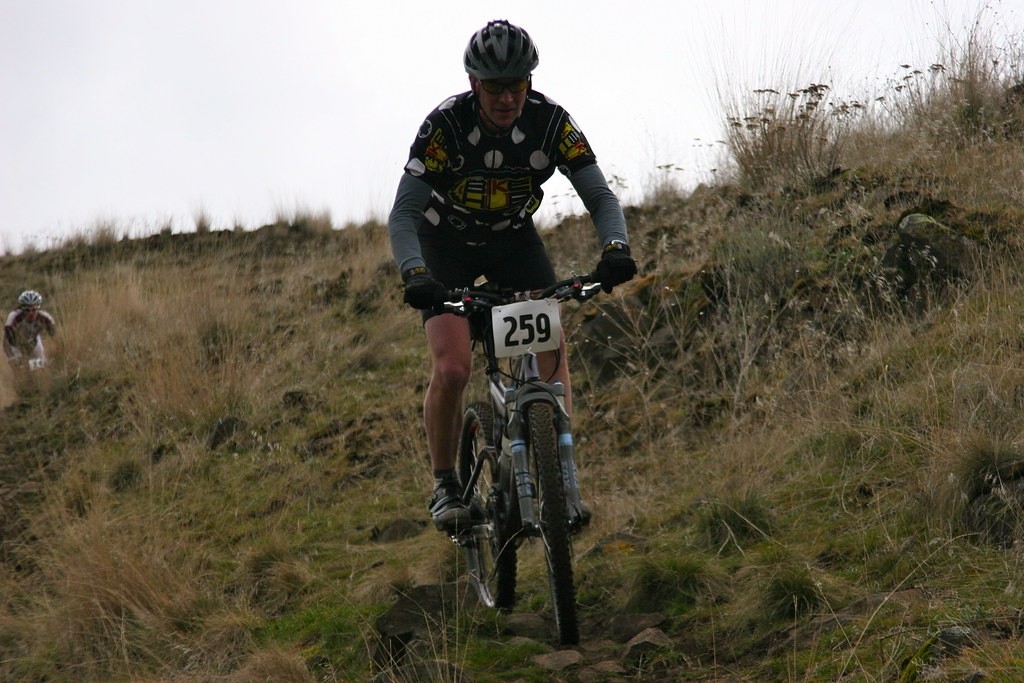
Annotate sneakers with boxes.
[429,478,471,532]
[538,496,593,526]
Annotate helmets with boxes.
[463,20,539,84]
[18,290,43,310]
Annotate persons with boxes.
[3,291,58,375]
[388,19,637,528]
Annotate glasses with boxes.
[473,77,530,95]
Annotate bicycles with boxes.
[403,267,608,648]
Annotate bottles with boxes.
[497,424,511,493]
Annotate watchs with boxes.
[605,240,625,246]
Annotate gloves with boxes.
[596,242,638,294]
[405,267,447,310]
